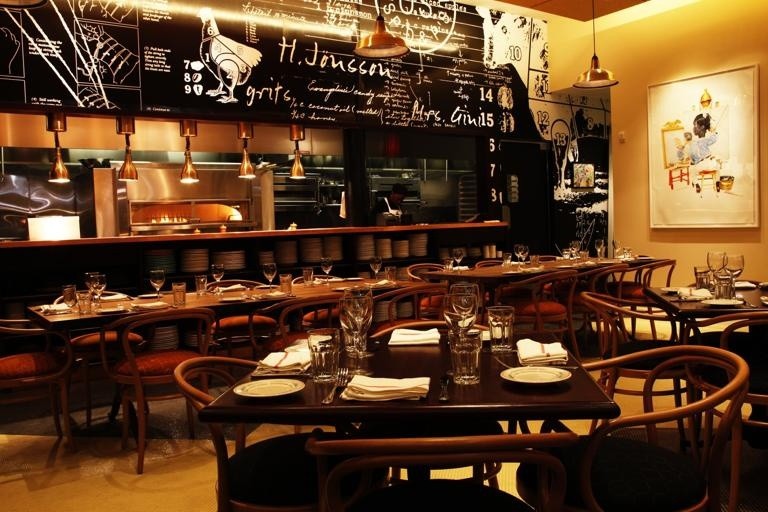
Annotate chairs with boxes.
[1,256,679,474]
[172,277,768,511]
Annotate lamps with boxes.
[116,116,138,180]
[354,0,410,59]
[237,121,256,179]
[180,119,200,183]
[290,123,305,179]
[47,110,71,183]
[573,0,619,88]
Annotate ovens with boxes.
[274,170,317,227]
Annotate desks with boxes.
[200,343,623,480]
[642,282,767,337]
[27,255,656,444]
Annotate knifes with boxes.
[437,373,450,401]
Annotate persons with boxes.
[689,113,717,165]
[370,183,407,224]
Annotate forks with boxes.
[323,366,350,404]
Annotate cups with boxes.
[303,266,314,290]
[279,273,294,298]
[195,275,207,296]
[449,330,482,382]
[169,282,186,305]
[487,305,514,354]
[385,265,396,286]
[308,327,340,385]
[79,289,93,314]
[63,286,76,309]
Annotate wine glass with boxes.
[263,262,275,293]
[209,262,225,297]
[441,240,632,273]
[83,270,93,287]
[338,284,373,377]
[443,284,481,376]
[368,256,382,282]
[90,273,106,310]
[148,270,164,304]
[695,249,744,299]
[319,255,333,288]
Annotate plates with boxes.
[374,298,413,324]
[659,287,696,294]
[146,231,428,277]
[236,377,304,400]
[499,365,573,385]
[284,343,310,353]
[150,324,214,353]
[700,299,745,307]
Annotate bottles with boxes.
[193,227,200,233]
[290,222,297,231]
[218,224,227,232]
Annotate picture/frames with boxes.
[647,62,760,229]
[570,161,595,189]
